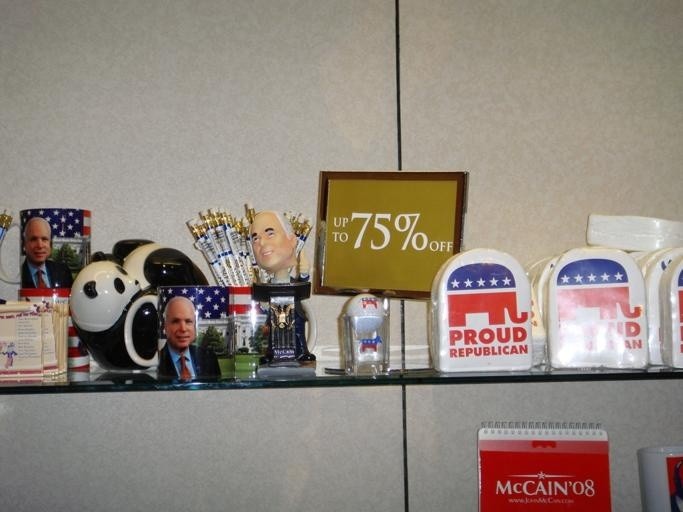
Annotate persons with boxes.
[18,217,74,287]
[154,296,222,383]
[247,208,300,283]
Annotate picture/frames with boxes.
[314,167,468,302]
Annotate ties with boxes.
[36,270,47,288]
[178,353,192,379]
